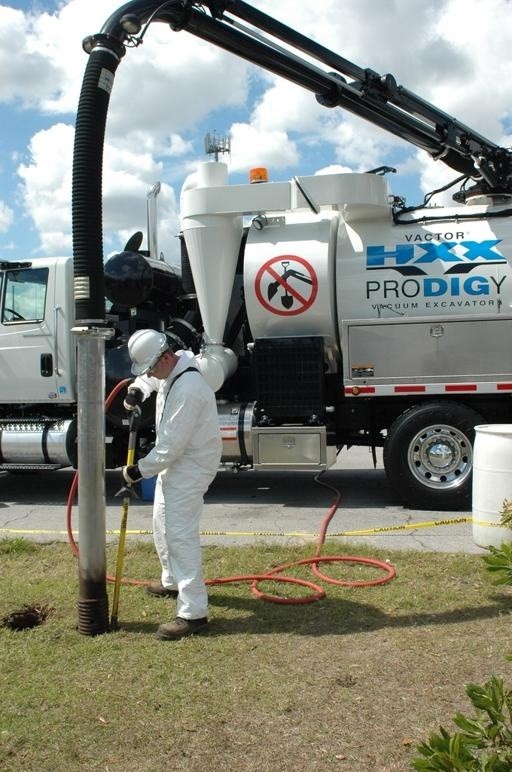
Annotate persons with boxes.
[122,327,223,640]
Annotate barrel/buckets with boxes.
[139,473,157,501]
[471,422,512,551]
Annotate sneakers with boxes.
[150,580,178,599]
[156,617,210,640]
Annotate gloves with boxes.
[113,467,143,496]
[122,387,145,418]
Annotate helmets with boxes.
[128,330,168,377]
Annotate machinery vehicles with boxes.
[0,0,512,636]
